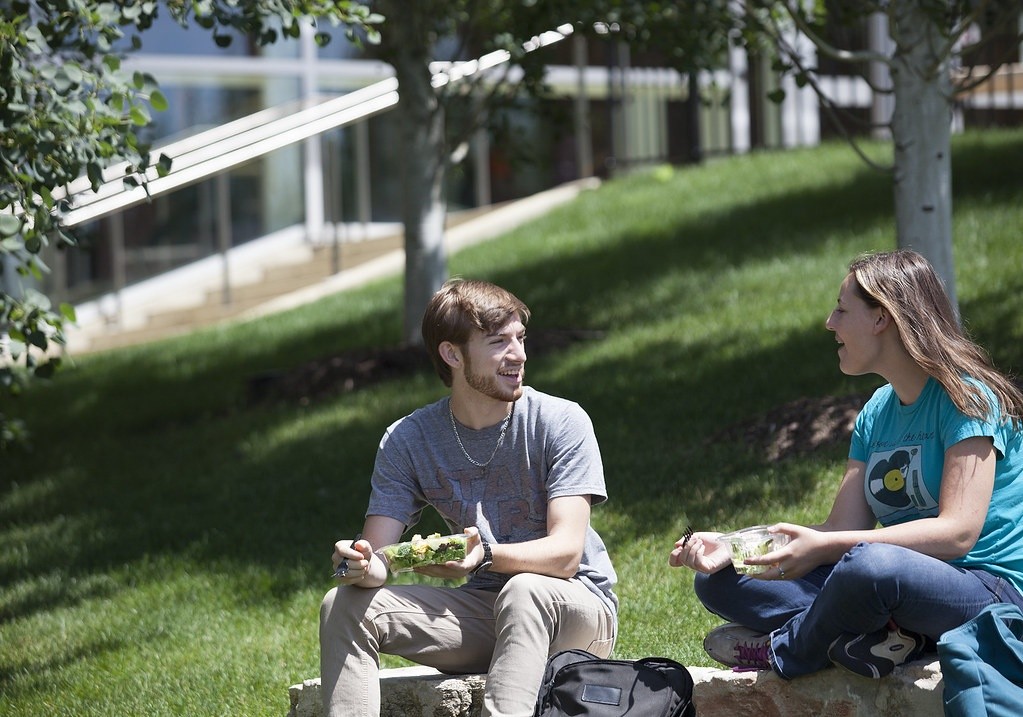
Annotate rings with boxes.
[778,566,785,580]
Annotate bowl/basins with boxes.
[375,534,471,574]
[718,525,791,574]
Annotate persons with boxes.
[316,277,621,716]
[665,249,1023,685]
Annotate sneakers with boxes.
[701,620,935,681]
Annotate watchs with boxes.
[470,541,494,576]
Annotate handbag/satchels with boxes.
[534,648,696,717]
[938,604,1023,717]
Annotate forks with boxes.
[331,534,362,577]
[680,525,694,548]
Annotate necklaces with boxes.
[447,397,513,467]
